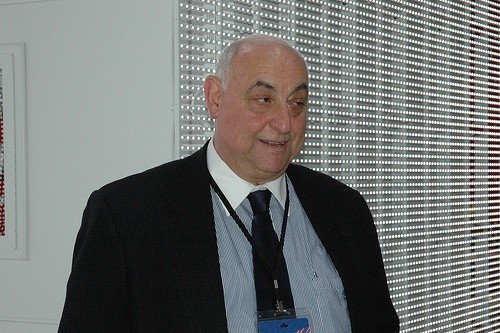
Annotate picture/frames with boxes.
[0,41,29,261]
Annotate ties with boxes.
[245,188,297,321]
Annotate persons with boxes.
[57,33,401,333]
